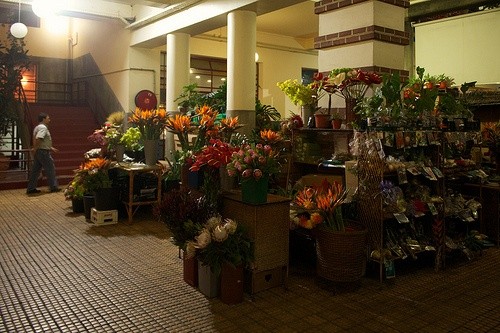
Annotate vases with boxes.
[183,253,198,287]
[143,139,159,166]
[241,176,268,205]
[219,264,246,305]
[116,144,125,163]
[314,113,332,129]
[346,97,360,127]
[301,104,311,128]
[198,258,219,297]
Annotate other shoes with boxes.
[27,189,41,194]
[51,188,62,192]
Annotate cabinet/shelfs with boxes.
[460,101,500,249]
[357,131,483,290]
[288,127,355,188]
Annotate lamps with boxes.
[10,0,28,39]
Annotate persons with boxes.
[26,112,64,194]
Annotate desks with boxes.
[221,189,292,295]
[116,167,161,224]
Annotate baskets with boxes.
[314,219,369,281]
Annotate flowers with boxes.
[63,67,500,273]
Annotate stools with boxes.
[89,207,118,227]
